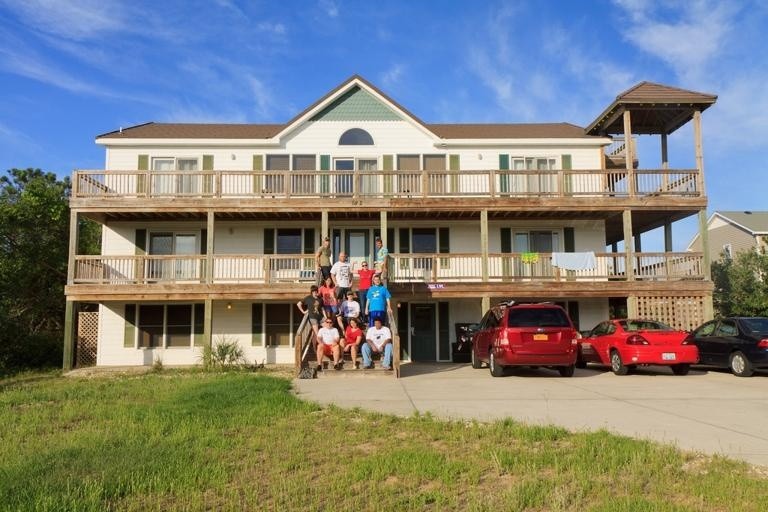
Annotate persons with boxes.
[297,237,393,369]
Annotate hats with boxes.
[347,291,352,295]
[325,237,330,241]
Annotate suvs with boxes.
[472,305,578,377]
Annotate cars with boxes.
[693,317,768,377]
[576,319,699,375]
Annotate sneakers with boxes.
[317,363,358,371]
